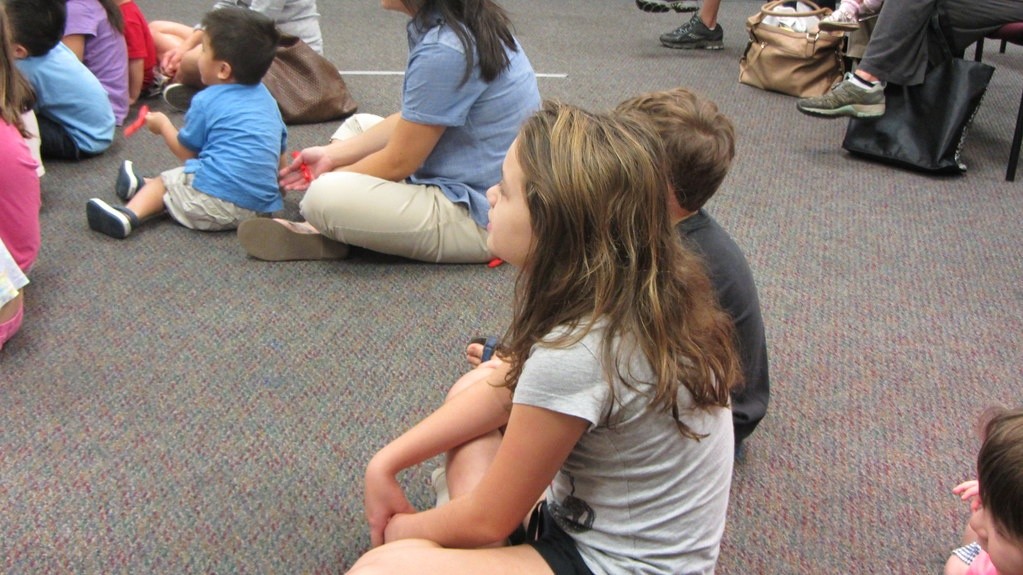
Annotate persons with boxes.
[635,0,1023,120]
[464,86,770,443]
[339,99,745,575]
[236,0,544,263]
[0,0,324,348]
[940,406,1022,575]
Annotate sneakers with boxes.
[796,72,887,118]
[636,0,698,13]
[659,11,725,49]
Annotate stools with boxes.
[974,21,1023,182]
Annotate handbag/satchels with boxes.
[263,25,355,123]
[738,0,845,99]
[840,12,995,176]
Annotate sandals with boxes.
[115,159,145,201]
[85,197,138,239]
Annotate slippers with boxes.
[237,218,347,261]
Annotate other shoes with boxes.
[466,336,508,363]
[818,0,877,32]
[146,66,197,112]
